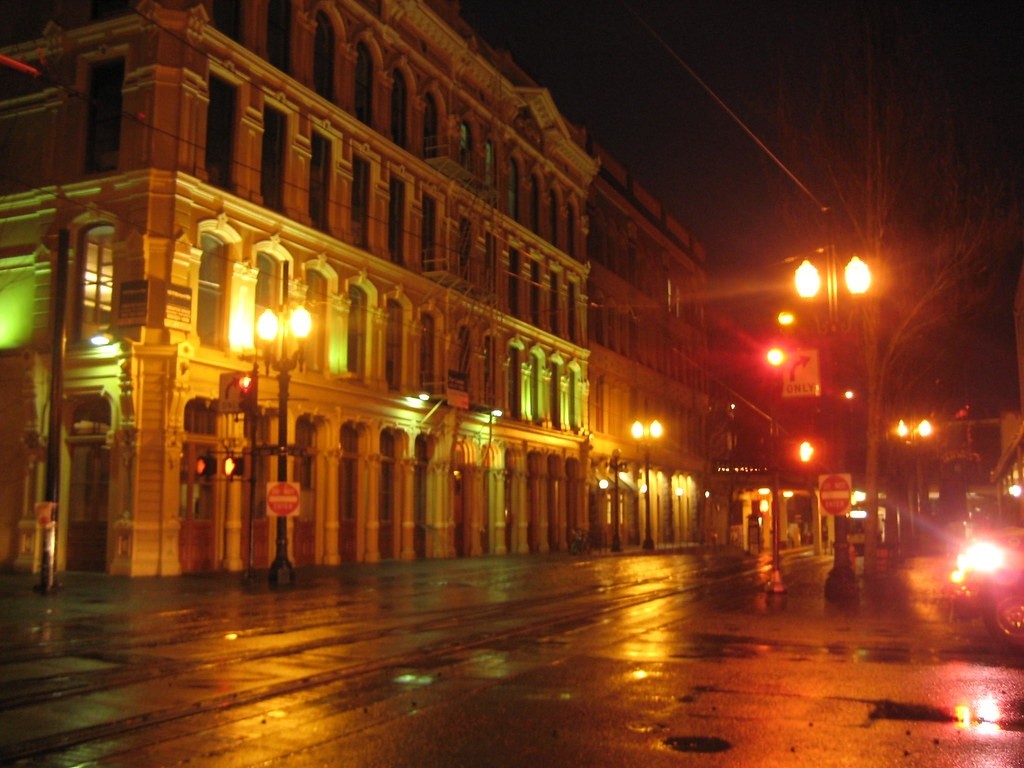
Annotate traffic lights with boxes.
[195,457,219,477]
[224,456,243,476]
[238,372,253,395]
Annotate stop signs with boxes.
[266,482,303,516]
[819,477,849,516]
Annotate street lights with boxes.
[256,303,311,587]
[630,418,663,552]
[896,417,934,555]
[790,255,875,606]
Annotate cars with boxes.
[950,525,1023,651]
